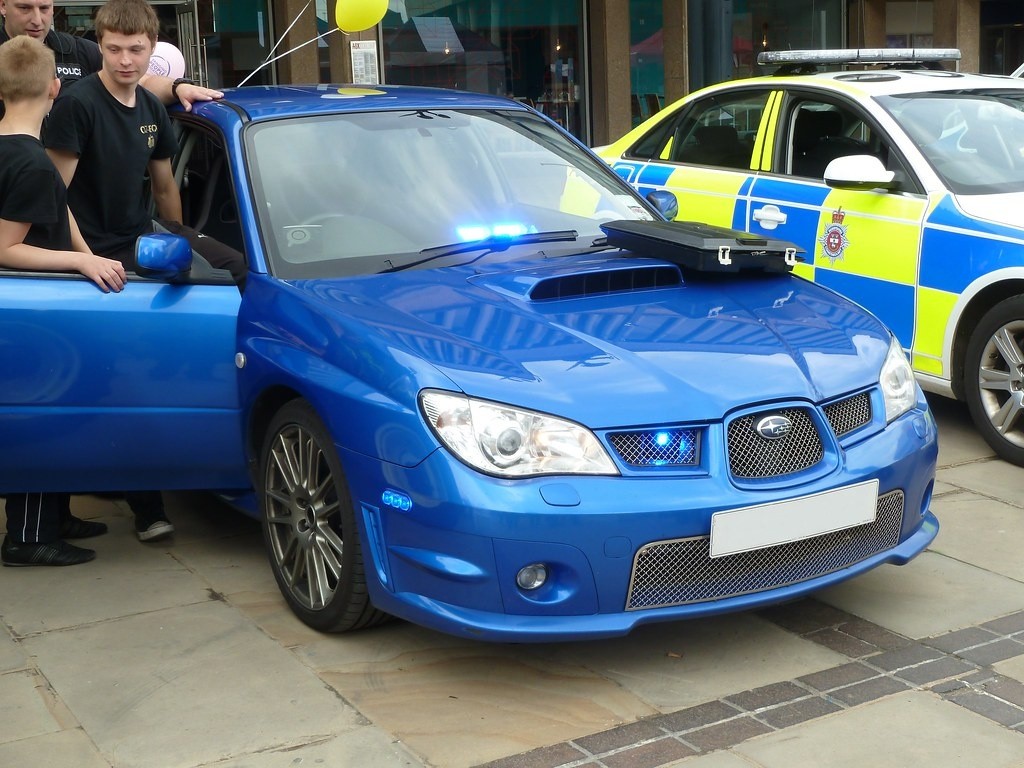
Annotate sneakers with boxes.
[136,515,176,541]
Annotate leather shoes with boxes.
[0,536,96,568]
[62,516,108,539]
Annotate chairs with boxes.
[677,101,963,194]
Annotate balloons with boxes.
[335,0,389,33]
[144,41,186,78]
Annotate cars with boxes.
[544,48,1024,465]
[1,84,940,643]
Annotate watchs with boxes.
[172,78,195,100]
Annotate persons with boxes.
[0,0,225,140]
[0,34,127,566]
[44,0,245,542]
